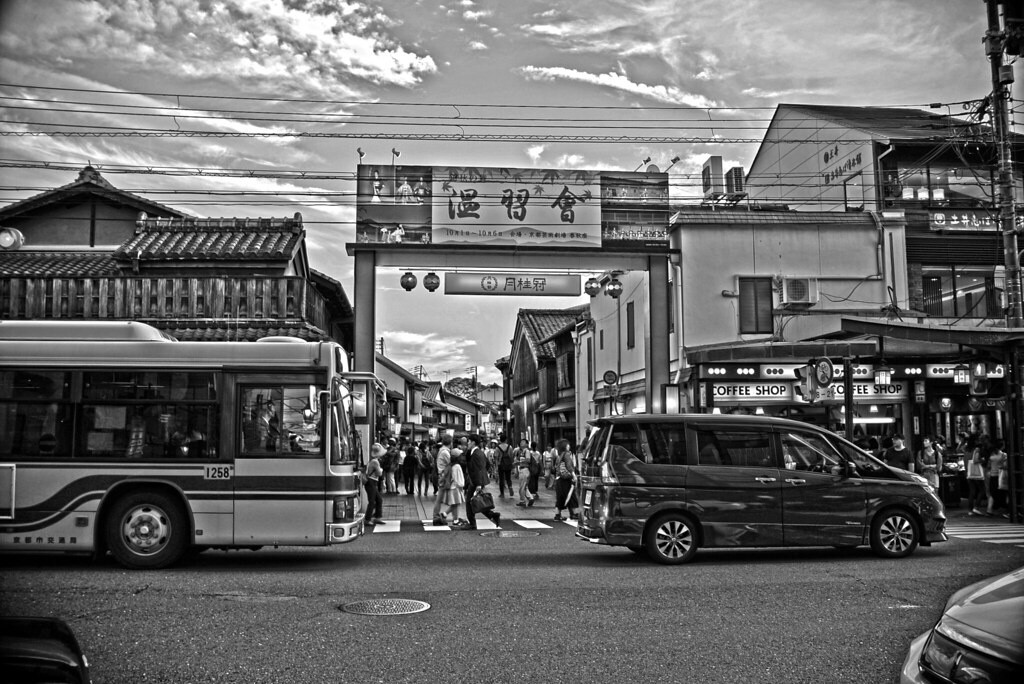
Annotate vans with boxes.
[576,412,948,564]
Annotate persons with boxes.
[364,433,581,531]
[758,432,1024,519]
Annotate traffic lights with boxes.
[970,362,988,396]
[793,365,810,401]
[0,228,25,251]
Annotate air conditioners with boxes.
[725,166,746,197]
[778,277,819,303]
[701,156,723,197]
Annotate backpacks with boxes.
[438,463,454,490]
[419,450,430,470]
[497,444,512,472]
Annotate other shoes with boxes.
[433,512,477,530]
[553,513,578,522]
[509,487,514,497]
[493,512,500,527]
[380,491,437,497]
[968,507,983,516]
[499,494,504,498]
[516,499,535,507]
[364,520,386,526]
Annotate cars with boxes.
[899,566,1024,684]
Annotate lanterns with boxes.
[400,272,623,298]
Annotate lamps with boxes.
[873,358,892,387]
[952,364,972,386]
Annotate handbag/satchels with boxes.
[362,469,375,485]
[470,490,494,513]
[558,453,572,480]
[529,455,541,476]
[967,460,985,480]
[998,468,1009,490]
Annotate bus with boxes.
[0,319,388,571]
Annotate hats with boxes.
[891,433,905,440]
[371,443,387,457]
[451,448,463,457]
